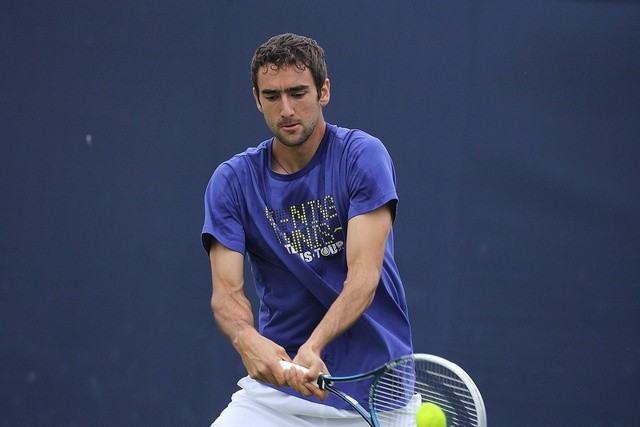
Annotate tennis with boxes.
[415,403,446,427]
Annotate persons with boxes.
[199,33,422,427]
